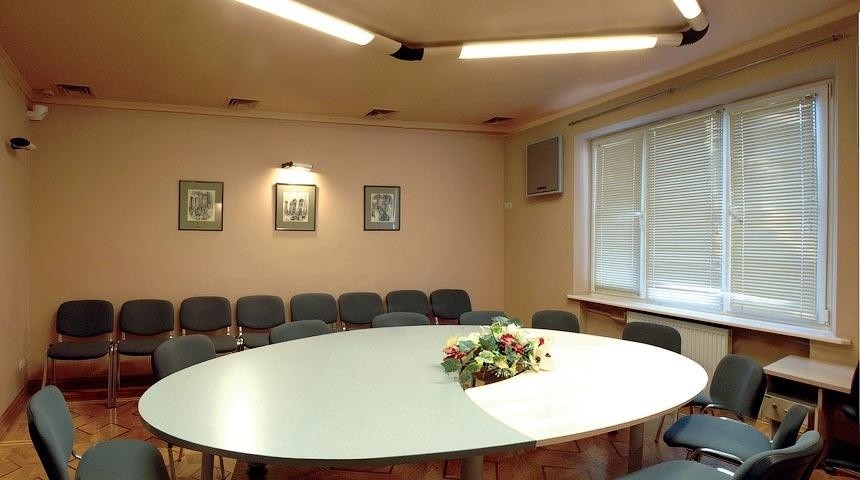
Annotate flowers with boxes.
[442,312,552,385]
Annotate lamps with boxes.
[233,0,409,63]
[410,32,689,65]
[671,0,714,42]
[281,160,313,172]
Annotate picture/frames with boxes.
[364,185,401,231]
[179,179,224,231]
[275,183,317,231]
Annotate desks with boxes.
[135,322,712,480]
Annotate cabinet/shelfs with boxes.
[761,354,856,476]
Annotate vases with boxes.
[459,365,538,398]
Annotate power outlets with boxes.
[18,358,26,372]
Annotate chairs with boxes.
[530,309,581,332]
[618,320,714,443]
[613,427,826,480]
[658,353,768,426]
[150,334,225,480]
[461,308,507,325]
[24,384,169,480]
[662,404,811,467]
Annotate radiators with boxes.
[623,310,733,406]
